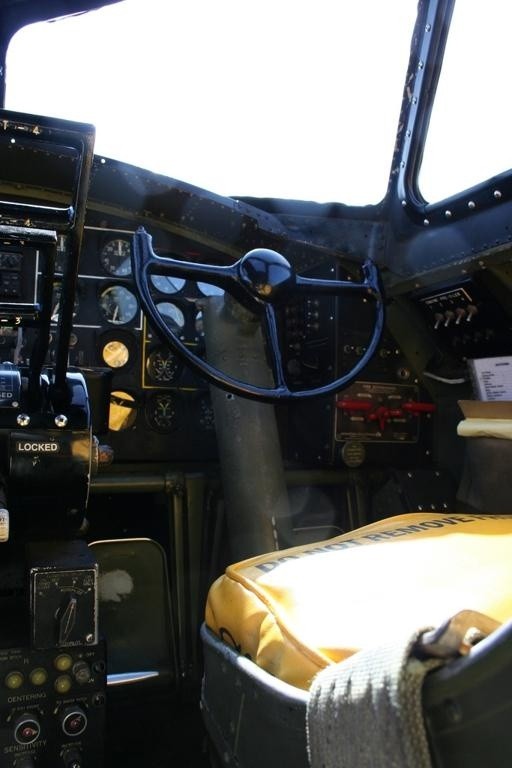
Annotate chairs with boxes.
[200,511,512,768]
